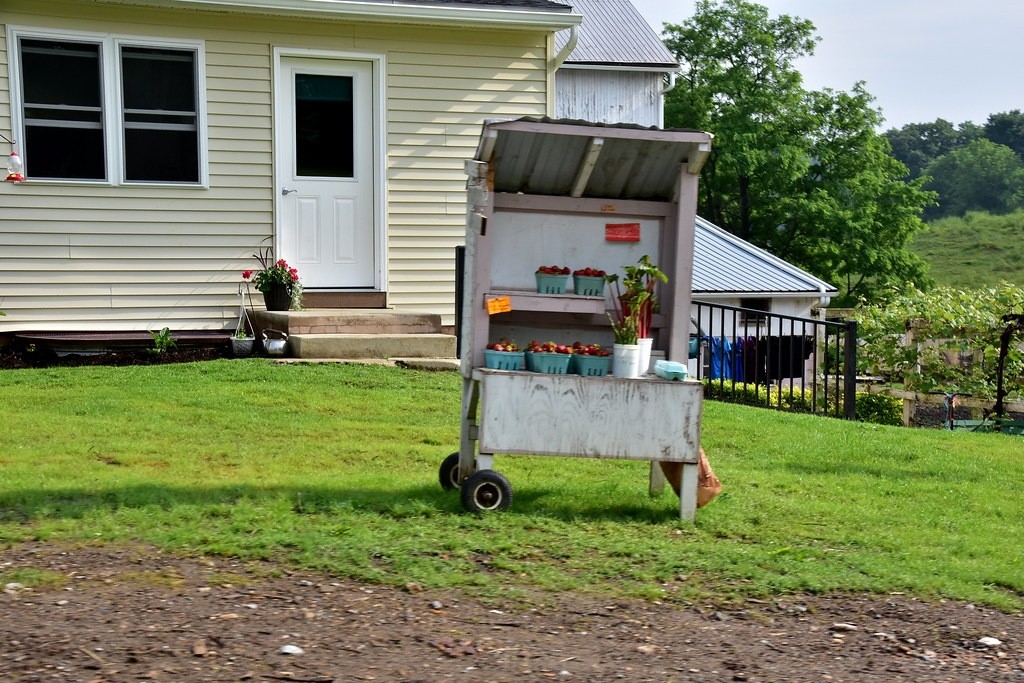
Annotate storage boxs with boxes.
[572,274,605,296]
[654,359,689,381]
[533,352,573,375]
[483,349,526,370]
[524,350,535,371]
[567,353,578,374]
[575,355,613,378]
[534,273,571,295]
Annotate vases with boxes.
[263,283,292,312]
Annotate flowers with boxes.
[242,259,309,313]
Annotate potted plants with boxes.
[607,290,651,377]
[606,252,669,376]
[231,328,256,358]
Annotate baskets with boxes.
[572,275,606,296]
[524,350,572,374]
[570,352,612,376]
[484,349,525,370]
[535,272,570,294]
